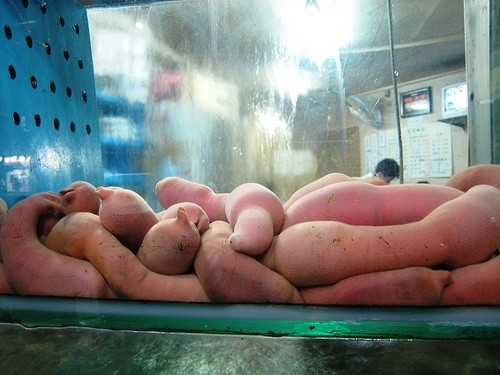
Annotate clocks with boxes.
[400,87,431,117]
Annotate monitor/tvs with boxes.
[443,81,467,116]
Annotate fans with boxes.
[346,95,383,129]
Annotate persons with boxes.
[358,159,400,186]
[144,70,193,213]
